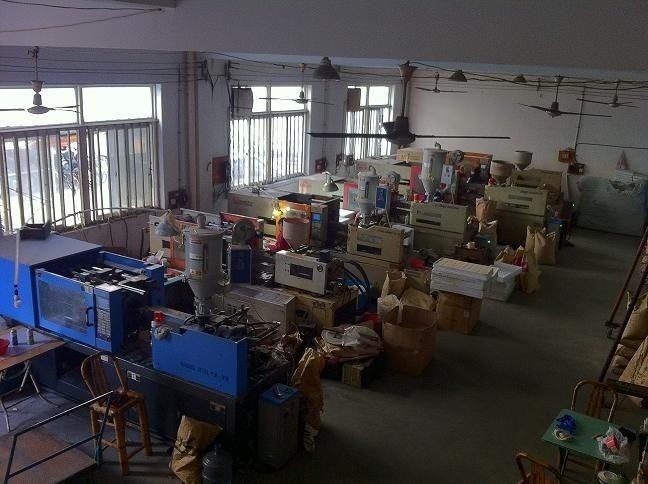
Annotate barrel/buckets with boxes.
[201,442,233,484]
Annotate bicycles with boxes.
[61,145,107,193]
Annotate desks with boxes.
[0,320,71,434]
[541,409,629,484]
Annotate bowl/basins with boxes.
[0,338,10,356]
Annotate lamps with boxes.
[321,172,346,192]
[311,56,342,81]
[446,65,469,82]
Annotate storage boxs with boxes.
[379,256,522,376]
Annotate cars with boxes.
[231,150,281,186]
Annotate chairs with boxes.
[556,376,623,483]
[78,347,155,477]
[513,450,569,483]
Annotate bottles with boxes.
[27,327,34,345]
[10,328,18,345]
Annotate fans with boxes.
[572,74,641,111]
[302,55,513,151]
[0,34,86,118]
[513,71,613,121]
[412,69,468,94]
[252,60,338,110]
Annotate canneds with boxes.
[26,329,34,345]
[10,329,17,344]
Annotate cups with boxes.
[468,241,475,249]
[196,214,206,229]
